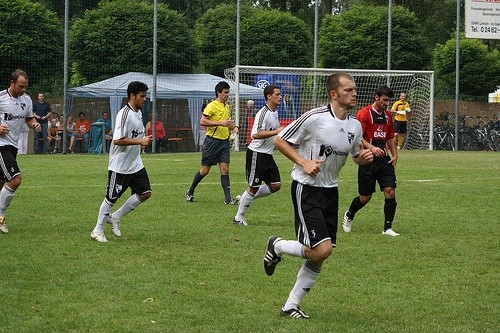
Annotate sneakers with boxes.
[224,199,239,205]
[0,216,9,233]
[90,230,109,243]
[342,210,354,233]
[186,189,194,203]
[280,305,310,319]
[382,228,401,236]
[109,213,123,236]
[233,217,248,226]
[263,235,284,276]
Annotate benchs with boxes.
[168,138,194,152]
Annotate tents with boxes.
[67,72,266,154]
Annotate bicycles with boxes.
[404,112,500,152]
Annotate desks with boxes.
[166,128,192,152]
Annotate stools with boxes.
[75,137,84,152]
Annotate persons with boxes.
[0,69,41,233]
[33,92,51,155]
[90,80,153,243]
[390,93,412,150]
[263,73,374,318]
[342,85,401,237]
[186,81,239,205]
[245,99,259,117]
[232,85,284,227]
[47,112,169,154]
